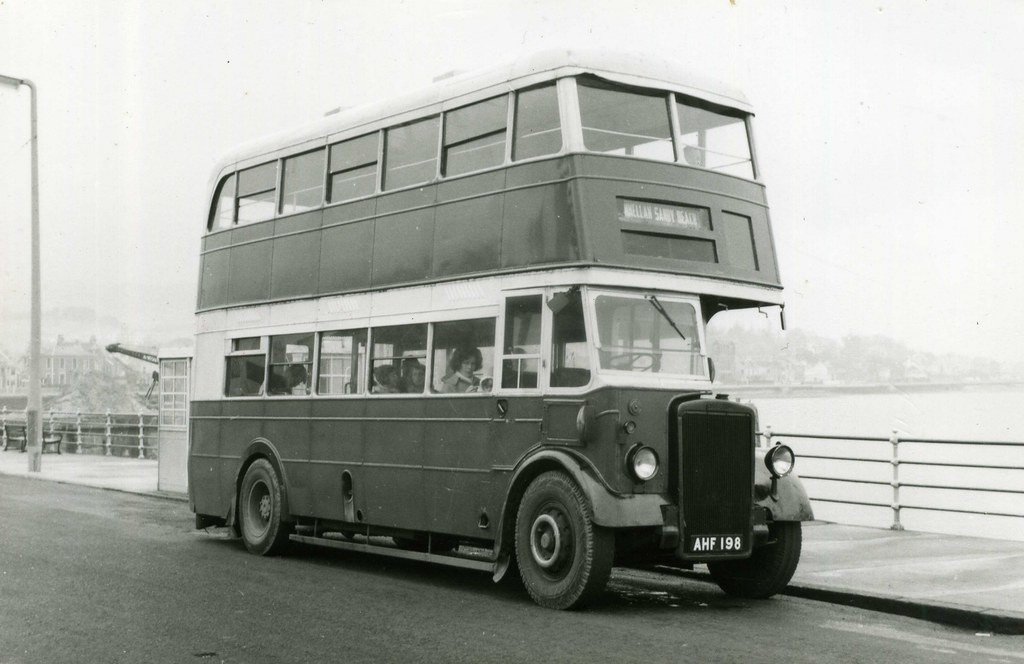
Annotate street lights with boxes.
[0,71,45,471]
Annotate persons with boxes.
[371,345,527,393]
[684,146,701,165]
[268,364,308,395]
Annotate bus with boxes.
[184,49,814,612]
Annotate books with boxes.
[466,377,495,393]
